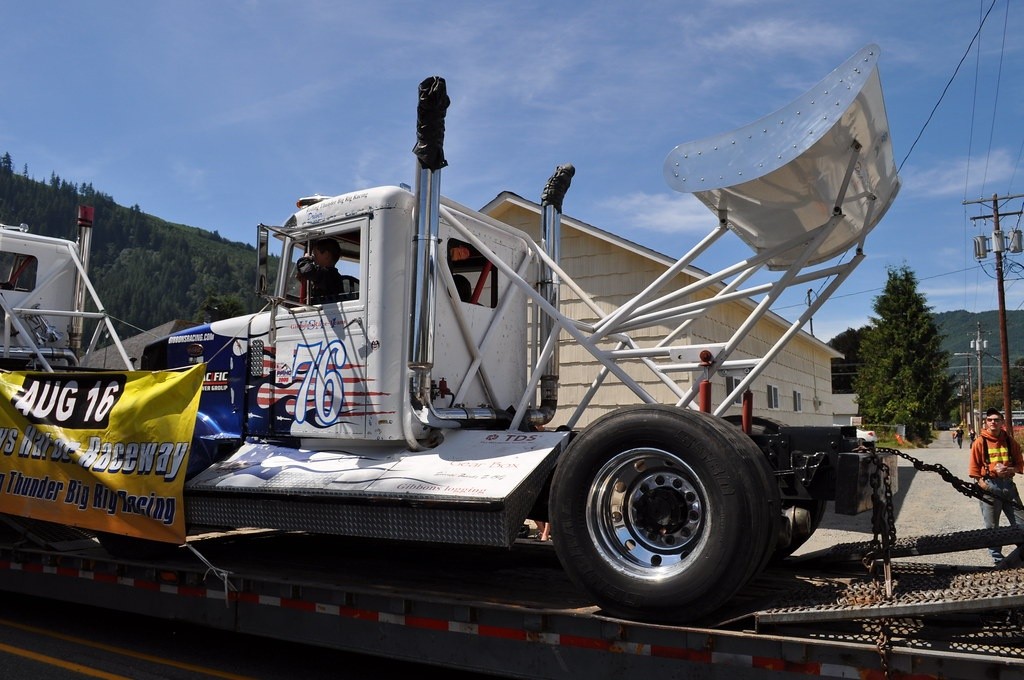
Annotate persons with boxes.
[968,423,976,449]
[952,426,964,449]
[297,238,348,305]
[974,411,1024,566]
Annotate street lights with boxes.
[954,353,982,435]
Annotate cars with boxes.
[833,424,877,443]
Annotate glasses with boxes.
[987,418,1002,422]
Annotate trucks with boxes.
[140,45,901,610]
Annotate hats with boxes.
[987,407,1004,420]
